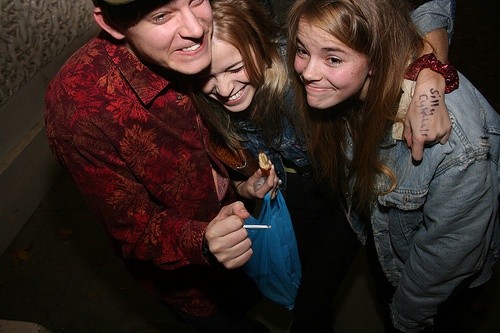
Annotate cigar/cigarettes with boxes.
[242,223,272,230]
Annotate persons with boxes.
[285,0,500,333]
[44,0,271,331]
[182,0,460,332]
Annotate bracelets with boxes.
[403,51,459,95]
